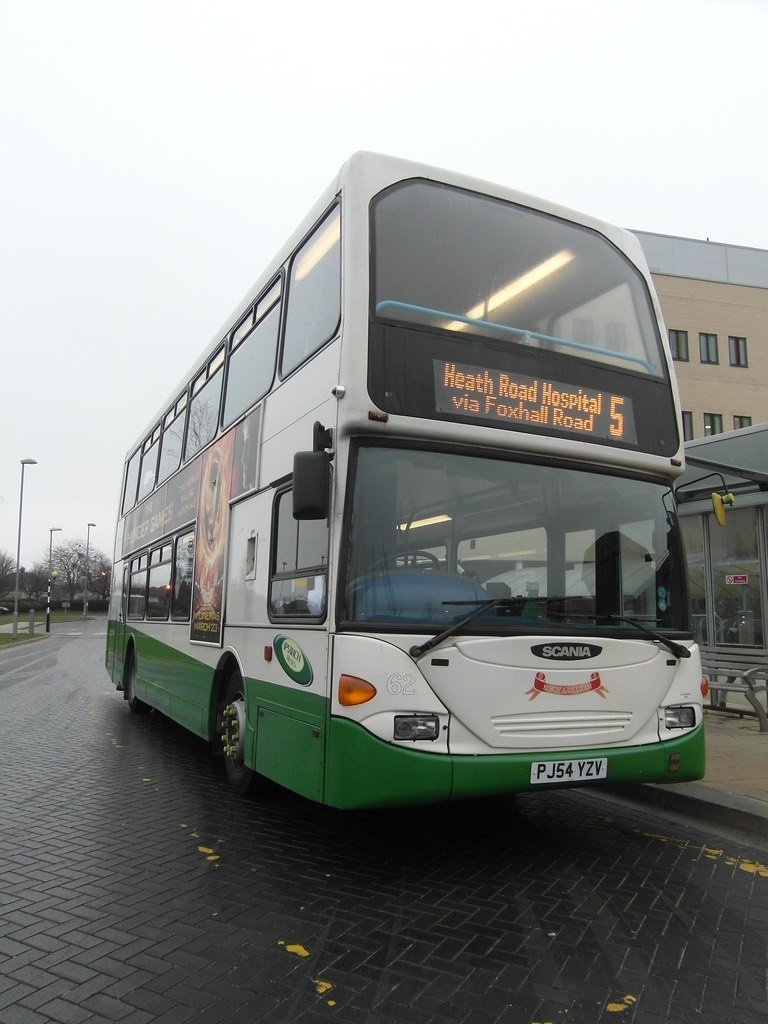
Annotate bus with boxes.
[105,151,736,813]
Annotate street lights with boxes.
[85,522,96,614]
[12,458,39,638]
[46,527,62,633]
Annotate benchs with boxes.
[695,647,768,730]
